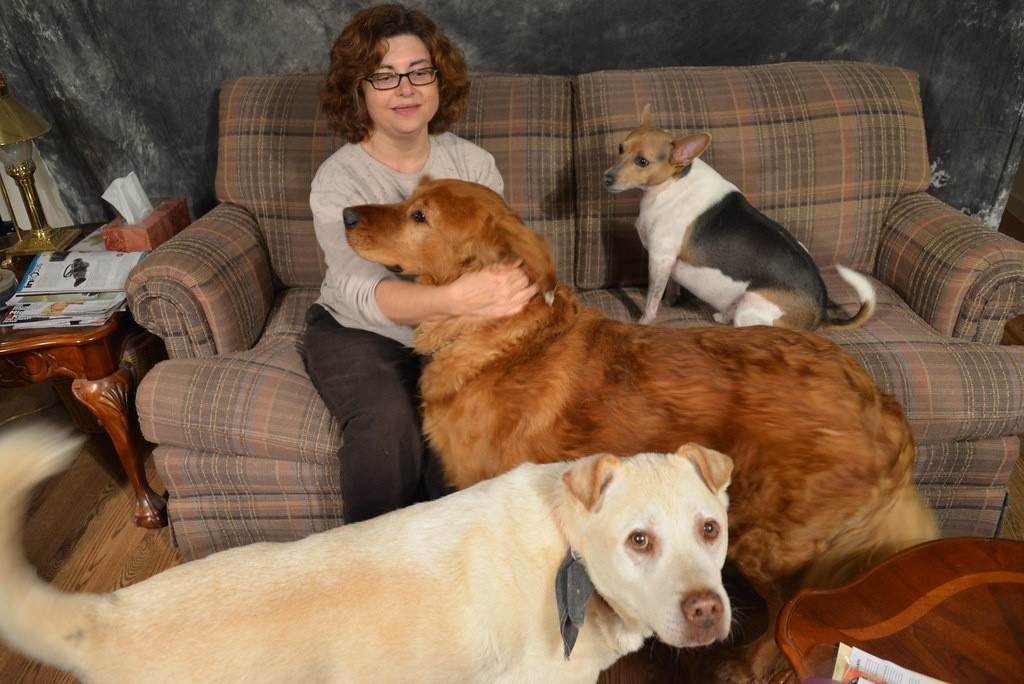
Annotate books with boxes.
[0,251,148,331]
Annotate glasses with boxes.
[363,67,439,90]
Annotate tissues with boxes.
[100,170,191,251]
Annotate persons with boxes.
[303,6,539,527]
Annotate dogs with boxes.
[601,103,877,335]
[0,421,737,684]
[342,173,942,684]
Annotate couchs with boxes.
[134,63,1024,565]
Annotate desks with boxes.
[0,222,169,528]
[771,537,1024,684]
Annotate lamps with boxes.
[0,74,82,254]
